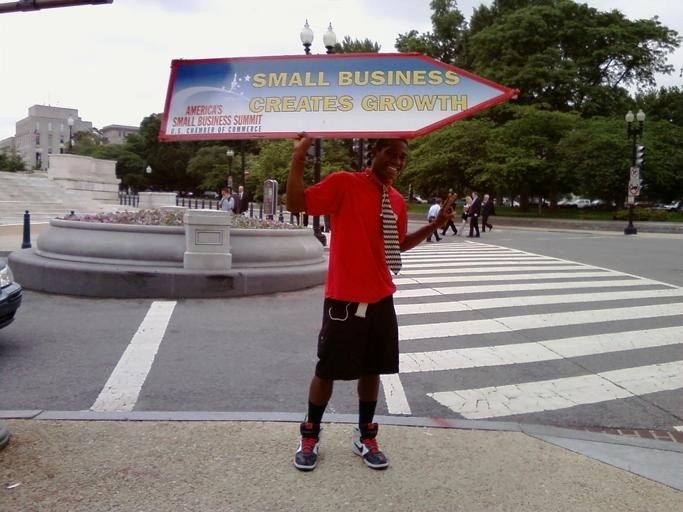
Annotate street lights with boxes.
[224,149,234,196]
[300,15,336,246]
[59,142,64,153]
[145,164,153,192]
[66,114,76,154]
[625,108,644,234]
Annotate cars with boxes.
[503,194,682,211]
[0,257,22,331]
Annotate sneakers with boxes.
[294,422,321,471]
[351,423,389,470]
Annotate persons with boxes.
[219,187,235,213]
[227,186,232,195]
[427,187,495,244]
[235,185,248,215]
[285,133,456,472]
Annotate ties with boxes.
[381,184,402,276]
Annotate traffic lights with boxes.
[635,144,644,169]
[362,138,375,168]
[350,159,360,170]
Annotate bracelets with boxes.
[432,219,444,229]
[291,151,306,163]
